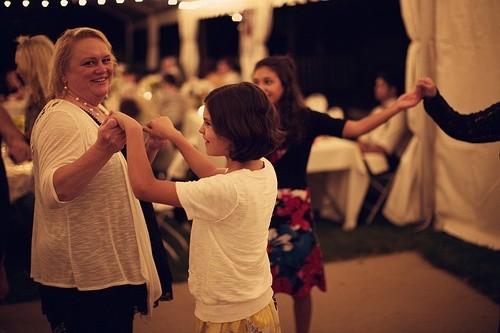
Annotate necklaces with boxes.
[76,96,104,120]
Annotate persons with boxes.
[0,36,405,299]
[109,82,287,333]
[253,56,422,333]
[30,27,172,333]
[418,77,500,143]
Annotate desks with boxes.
[303,136,371,231]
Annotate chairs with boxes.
[362,129,416,228]
